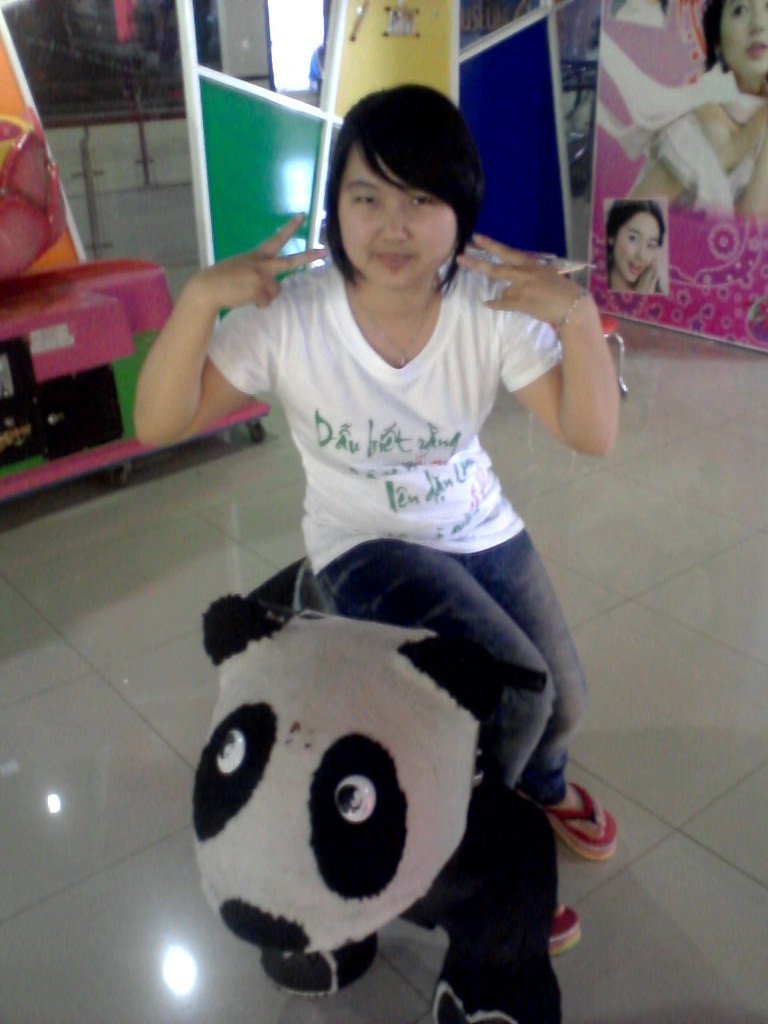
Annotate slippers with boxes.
[548,906,582,956]
[517,781,618,861]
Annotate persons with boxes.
[627,0,768,218]
[132,84,621,962]
[606,199,665,293]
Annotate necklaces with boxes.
[355,281,437,368]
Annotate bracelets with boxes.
[561,288,592,326]
